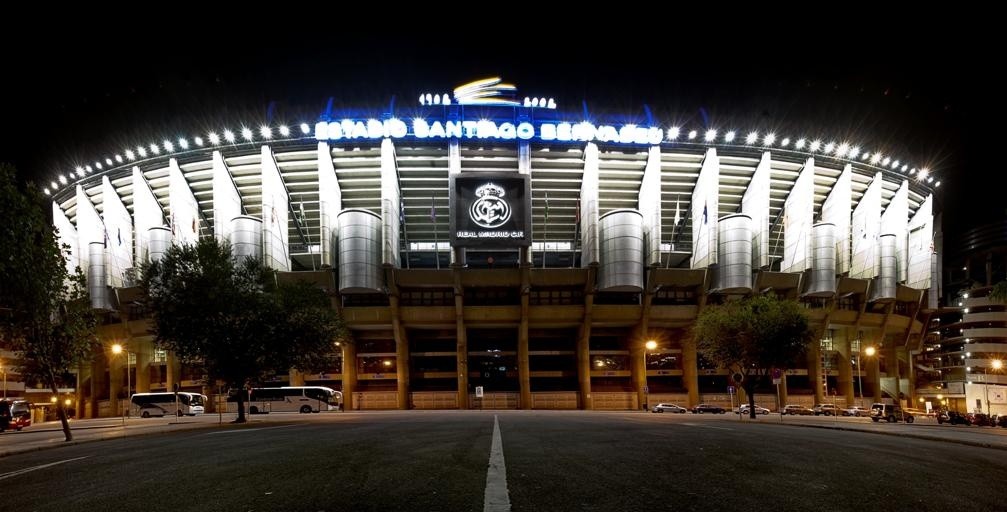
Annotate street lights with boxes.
[857,348,874,407]
[486,348,502,409]
[334,340,345,395]
[642,340,657,412]
[985,361,1001,416]
[111,343,130,417]
[0,359,8,399]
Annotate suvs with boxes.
[935,410,1006,427]
[813,403,848,417]
[869,403,913,422]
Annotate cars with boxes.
[735,404,770,415]
[846,405,870,416]
[784,404,814,415]
[691,404,726,414]
[651,402,687,413]
[903,407,934,420]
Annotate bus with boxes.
[128,391,208,418]
[223,385,342,413]
[0,398,36,430]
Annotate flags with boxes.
[673,200,680,227]
[860,222,866,239]
[299,202,305,226]
[171,212,175,237]
[271,205,276,229]
[430,199,437,219]
[703,202,707,225]
[117,228,122,247]
[192,216,196,233]
[928,239,934,256]
[575,202,579,222]
[398,200,405,223]
[101,226,109,249]
[543,198,550,219]
